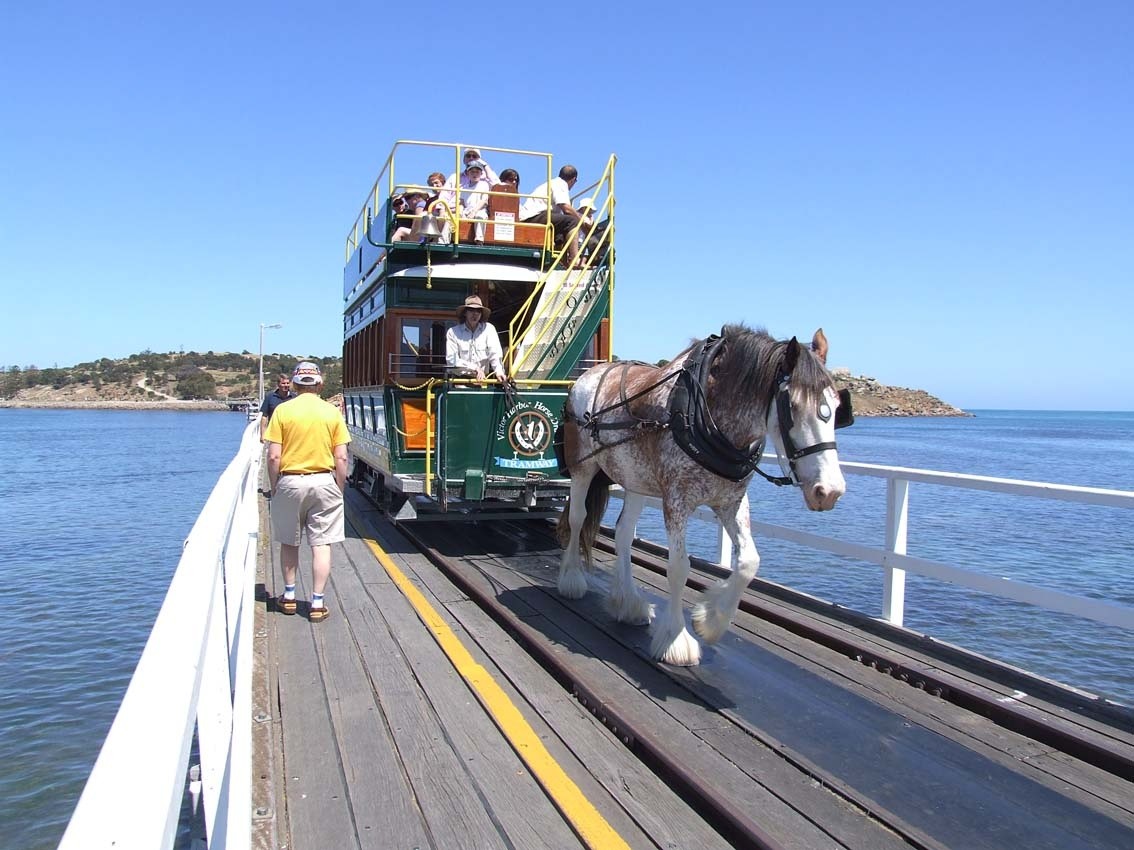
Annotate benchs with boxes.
[410,217,545,247]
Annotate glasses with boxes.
[466,307,482,313]
[404,195,413,201]
[466,153,479,158]
[393,199,403,207]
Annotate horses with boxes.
[557,320,846,667]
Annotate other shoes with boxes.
[476,241,484,248]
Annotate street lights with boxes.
[257,323,283,410]
[261,371,271,401]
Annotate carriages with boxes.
[339,138,857,666]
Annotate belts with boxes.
[282,470,330,475]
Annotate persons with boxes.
[392,147,604,271]
[259,360,354,623]
[444,293,510,388]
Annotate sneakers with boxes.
[277,593,299,614]
[309,606,328,622]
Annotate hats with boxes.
[463,147,482,165]
[403,185,429,202]
[393,192,409,213]
[293,362,321,385]
[456,295,491,322]
[576,198,597,214]
[466,160,483,171]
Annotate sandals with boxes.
[566,257,597,269]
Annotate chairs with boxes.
[488,183,519,222]
